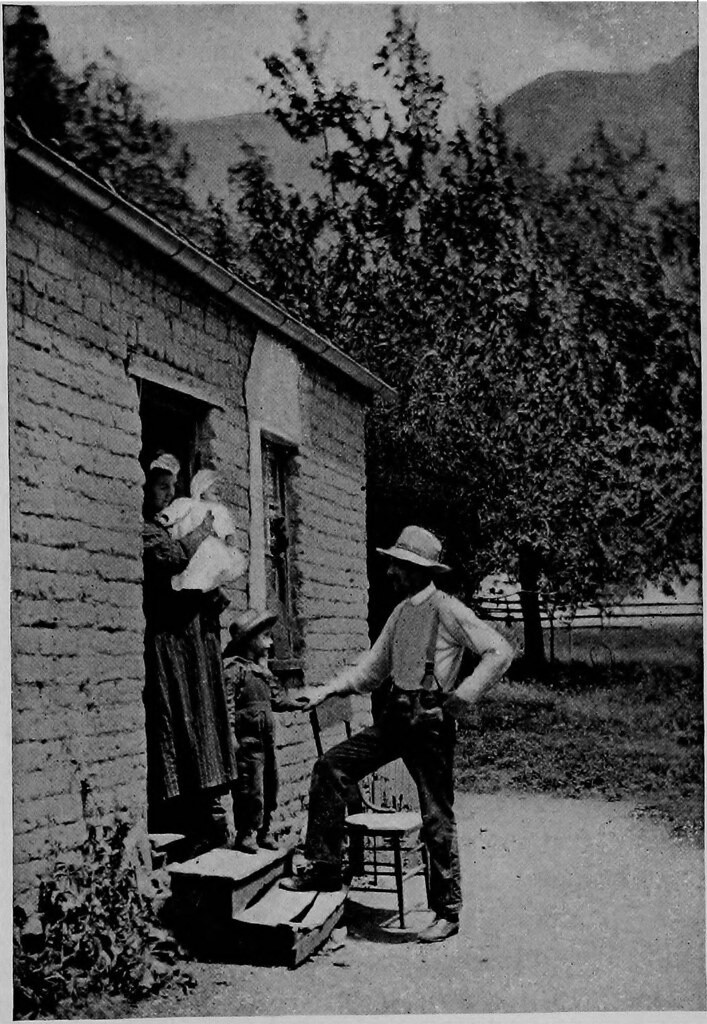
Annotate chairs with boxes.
[310,692,431,929]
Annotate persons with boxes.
[223,610,309,853]
[282,525,515,943]
[143,449,237,845]
[158,468,248,610]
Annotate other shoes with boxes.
[256,835,279,850]
[236,836,260,852]
[417,919,460,942]
[279,864,343,891]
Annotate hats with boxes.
[225,610,278,654]
[376,526,450,572]
[190,469,219,499]
